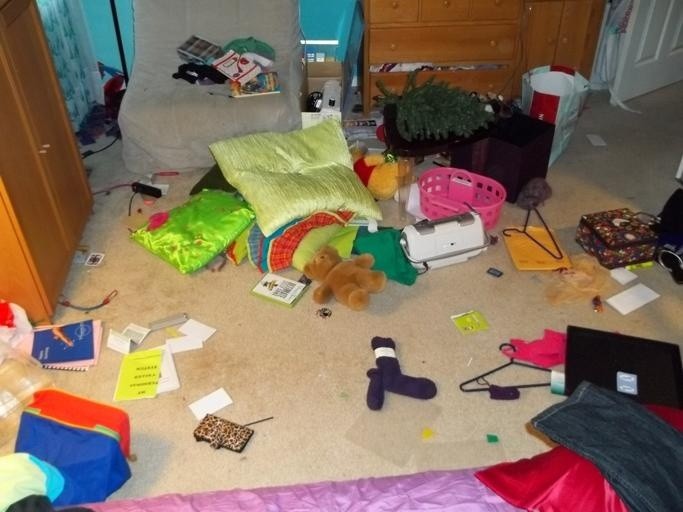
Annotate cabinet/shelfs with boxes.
[523,1,606,80]
[0,1,96,325]
[362,0,524,113]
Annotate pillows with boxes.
[206,119,384,238]
[475,403,682,511]
[129,189,256,275]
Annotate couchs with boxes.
[117,1,310,175]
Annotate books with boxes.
[229,72,281,98]
[213,48,261,87]
[249,272,310,309]
[31,319,103,371]
[177,36,219,63]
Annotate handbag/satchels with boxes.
[522,65,591,169]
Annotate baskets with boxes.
[418,166,506,232]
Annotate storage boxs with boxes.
[305,62,348,104]
[484,115,555,203]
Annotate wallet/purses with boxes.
[193,414,254,452]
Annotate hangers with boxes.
[503,207,563,259]
[459,343,551,393]
